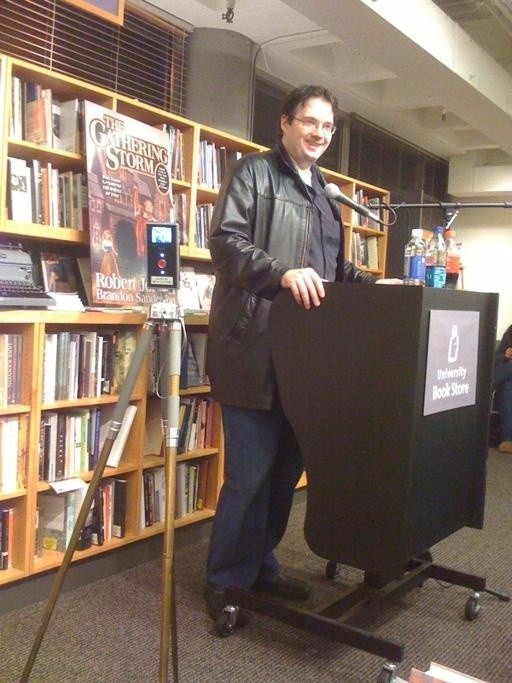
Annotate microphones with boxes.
[324,182,381,223]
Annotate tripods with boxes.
[20,300,184,683]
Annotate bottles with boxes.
[402,226,461,290]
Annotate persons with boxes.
[496,323,511,453]
[199,77,401,644]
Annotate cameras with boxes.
[145,223,181,290]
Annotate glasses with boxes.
[287,114,337,135]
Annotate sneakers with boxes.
[204,580,247,627]
[250,568,311,601]
[499,441,512,454]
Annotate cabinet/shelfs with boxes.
[0,51,391,586]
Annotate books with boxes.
[199,141,242,193]
[353,189,381,270]
[141,333,214,530]
[10,78,87,156]
[155,123,185,182]
[194,203,215,247]
[177,265,217,314]
[0,333,23,569]
[34,331,131,552]
[42,251,103,312]
[172,194,188,244]
[7,156,90,231]
